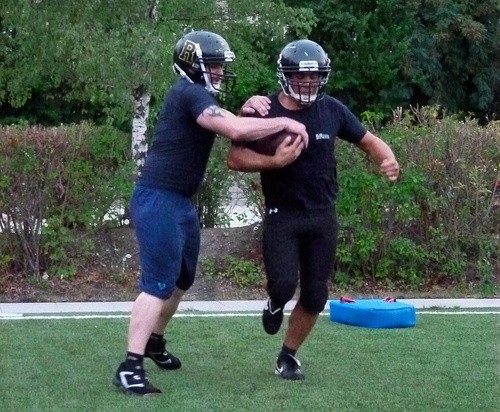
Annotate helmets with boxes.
[173,31,236,95]
[276,40,331,106]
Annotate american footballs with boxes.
[245,129,304,156]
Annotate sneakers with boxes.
[112,361,161,397]
[144,338,181,370]
[262,296,284,335]
[274,353,305,380]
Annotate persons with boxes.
[111,30,308,396]
[228,40,399,380]
